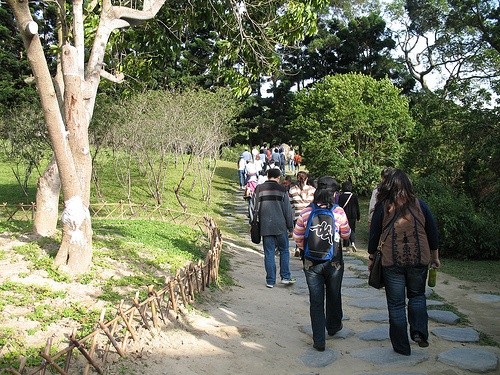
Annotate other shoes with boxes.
[295,249,300,257]
[274,250,280,255]
[313,342,325,351]
[413,334,429,348]
[288,234,293,238]
[352,244,357,252]
[346,250,350,254]
[328,323,343,335]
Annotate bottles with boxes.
[428,267,436,287]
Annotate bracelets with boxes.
[369,256,374,261]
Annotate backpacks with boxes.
[245,164,256,175]
[304,203,340,261]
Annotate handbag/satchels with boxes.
[368,251,384,289]
[251,221,260,244]
[243,185,253,199]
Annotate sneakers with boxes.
[281,278,297,284]
[267,282,273,288]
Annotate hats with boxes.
[247,176,258,183]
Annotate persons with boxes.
[287,171,316,257]
[368,168,391,227]
[368,169,440,355]
[338,182,360,252]
[247,168,296,288]
[237,142,295,219]
[293,176,351,351]
